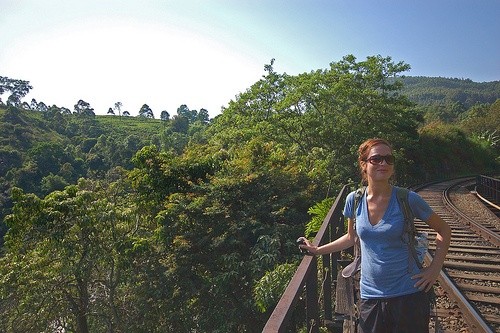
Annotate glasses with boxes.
[363,154,395,166]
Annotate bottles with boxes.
[415,231,429,264]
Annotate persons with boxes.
[297,135,452,333]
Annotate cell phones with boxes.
[296,239,309,255]
